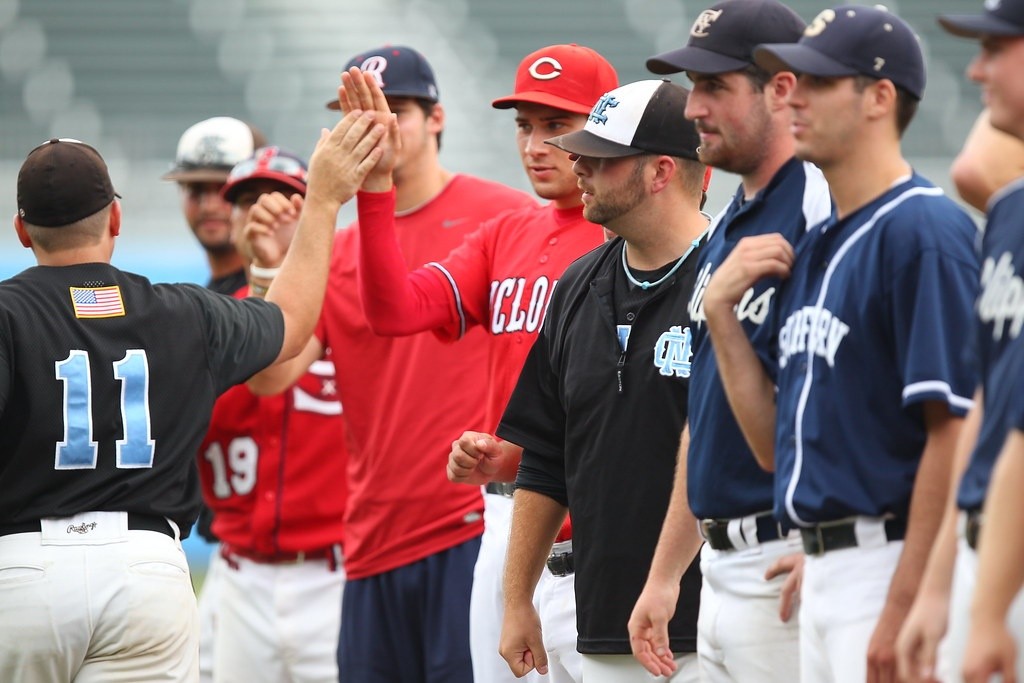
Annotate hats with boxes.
[936,0,1024,40]
[220,146,310,202]
[492,43,620,116]
[159,116,268,185]
[645,0,809,75]
[751,3,926,102]
[543,78,700,162]
[326,42,439,111]
[16,136,123,228]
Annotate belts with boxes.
[546,551,575,577]
[701,513,800,553]
[0,511,176,540]
[965,509,981,550]
[486,481,517,495]
[227,544,334,562]
[800,518,907,556]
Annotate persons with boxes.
[339,0,1023,683]
[0,42,545,683]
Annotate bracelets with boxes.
[249,261,282,296]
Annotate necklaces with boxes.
[621,212,714,289]
